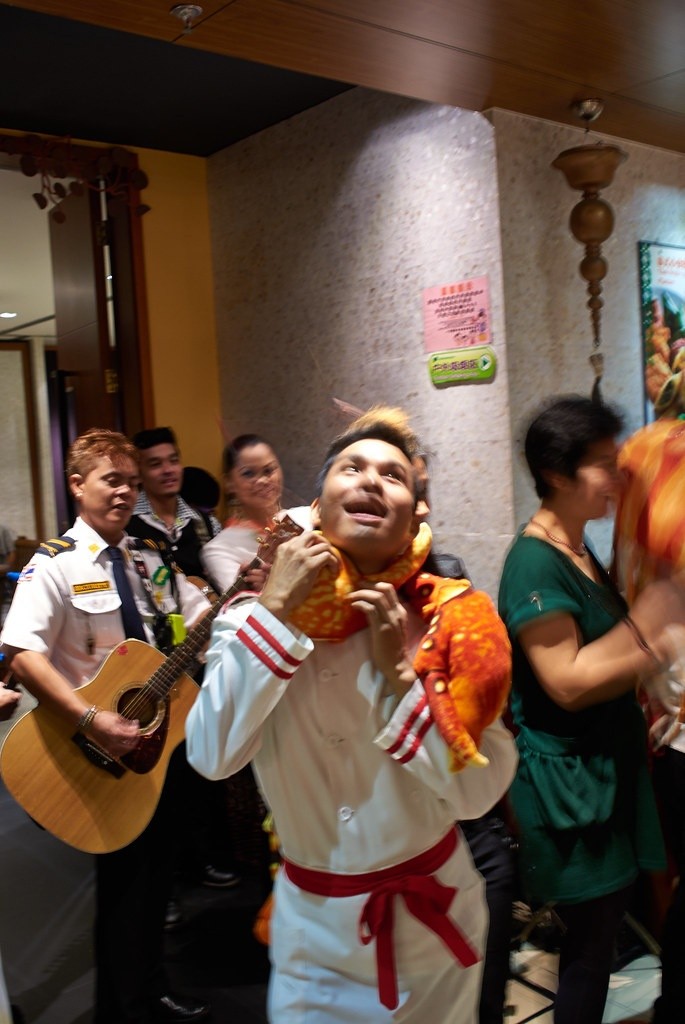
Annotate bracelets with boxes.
[622,614,656,660]
[79,704,101,731]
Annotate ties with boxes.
[105,545,152,656]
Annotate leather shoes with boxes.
[154,991,214,1022]
[165,899,183,928]
[179,860,237,886]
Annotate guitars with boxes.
[0,512,308,859]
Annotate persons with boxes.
[185,404,519,1024]
[0,395,685,1024]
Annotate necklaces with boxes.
[530,517,588,558]
